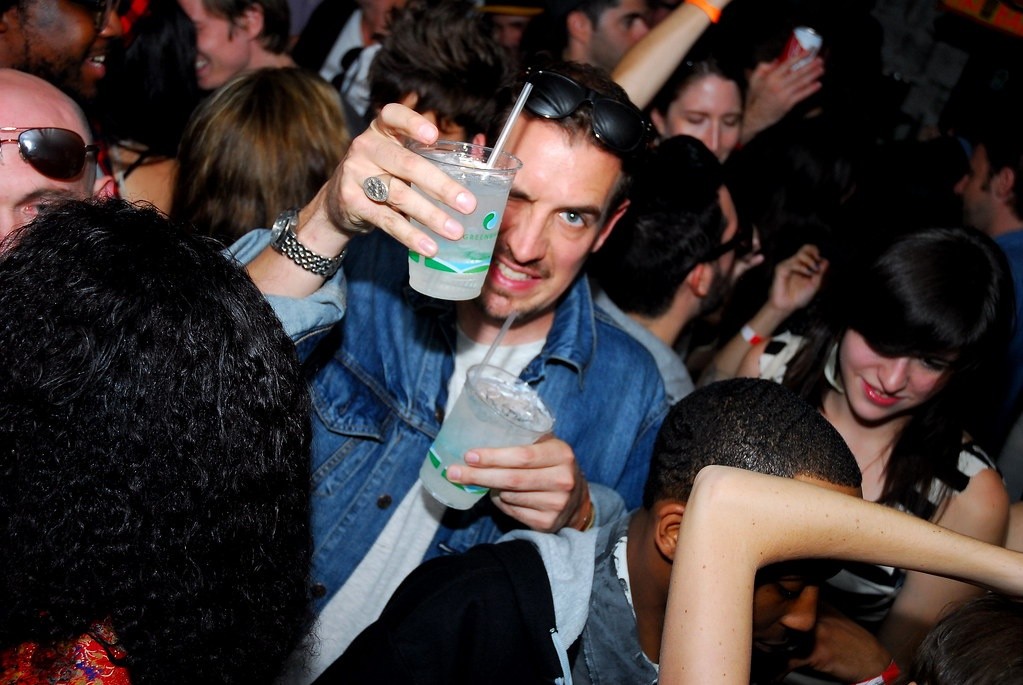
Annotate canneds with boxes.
[777,27,822,76]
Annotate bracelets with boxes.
[581,502,595,532]
[685,0,722,23]
[740,324,764,346]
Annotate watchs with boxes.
[270,208,345,278]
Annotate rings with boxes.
[364,174,393,203]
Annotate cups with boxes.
[420,364,554,510]
[409,141,523,301]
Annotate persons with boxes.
[0,0,1023,685]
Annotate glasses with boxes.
[67,0,134,31]
[695,222,741,268]
[488,65,653,174]
[330,46,365,97]
[0,125,102,182]
